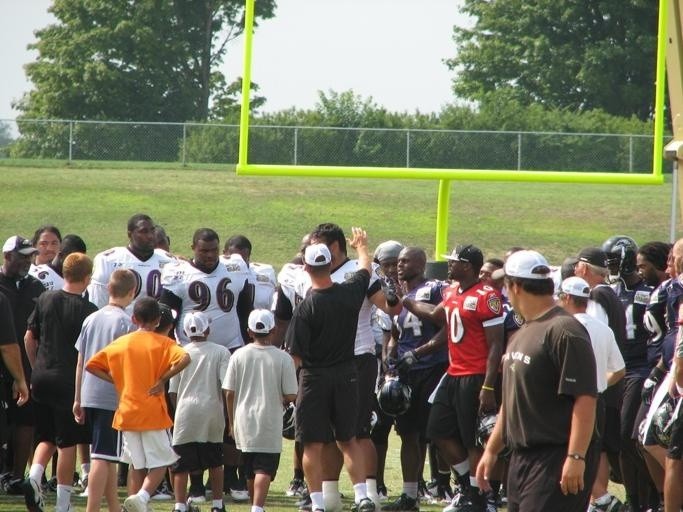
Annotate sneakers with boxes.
[0,475,251,502]
[381,493,419,512]
[586,490,683,511]
[22,480,44,512]
[286,480,389,512]
[420,478,498,512]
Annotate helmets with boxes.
[473,409,514,459]
[601,235,640,283]
[283,404,300,441]
[376,377,412,419]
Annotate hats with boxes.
[555,276,592,299]
[248,309,276,334]
[490,249,552,281]
[441,243,483,265]
[563,247,608,268]
[184,311,211,337]
[2,235,40,255]
[305,243,332,266]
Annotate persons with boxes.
[0,212,683,512]
